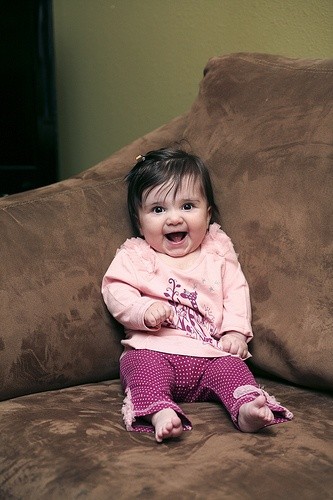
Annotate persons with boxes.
[99,144,276,443]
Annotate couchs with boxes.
[0,51,332,500]
